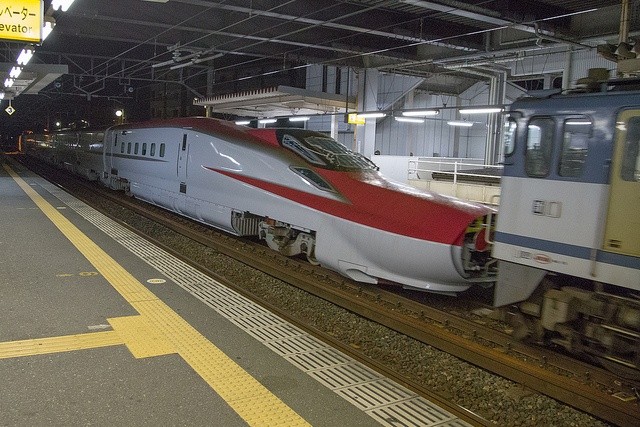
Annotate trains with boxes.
[490,77,640,380]
[19,116,498,297]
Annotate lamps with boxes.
[259,118,278,124]
[27,17,58,47]
[357,112,387,118]
[235,120,250,125]
[3,78,15,88]
[459,108,504,114]
[447,120,474,127]
[401,110,439,116]
[289,117,310,121]
[16,45,36,66]
[0,90,5,99]
[9,66,24,78]
[50,0,74,12]
[395,116,425,123]
